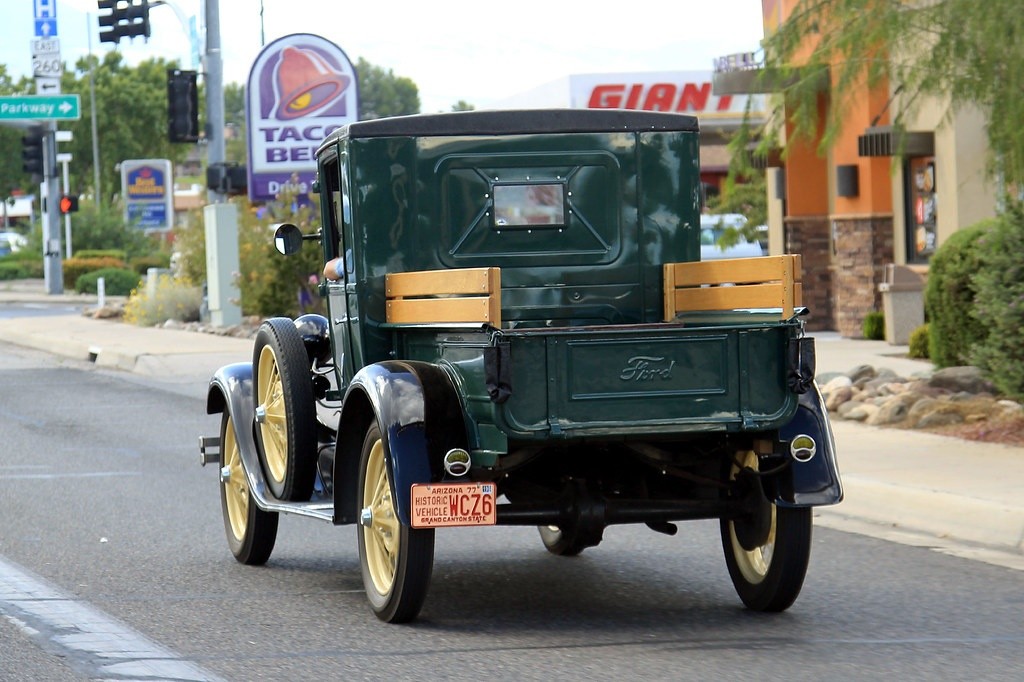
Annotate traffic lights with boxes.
[114,0,136,41]
[130,2,152,44]
[21,124,51,184]
[97,0,118,44]
[59,195,77,214]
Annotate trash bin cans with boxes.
[879,263,924,347]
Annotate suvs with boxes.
[198,104,850,627]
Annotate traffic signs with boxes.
[0,94,82,124]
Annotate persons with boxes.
[323,256,345,279]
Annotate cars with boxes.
[697,213,771,278]
